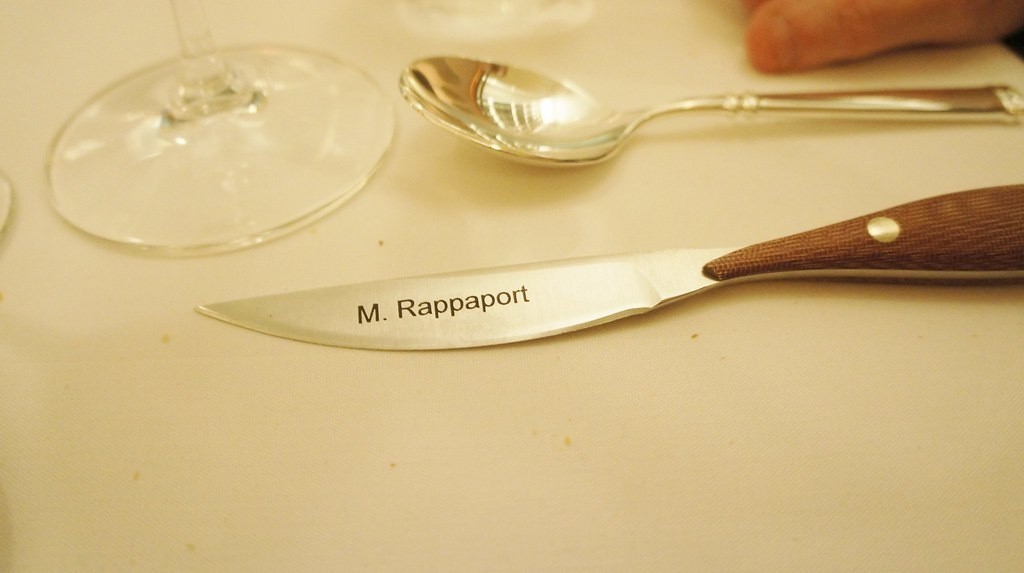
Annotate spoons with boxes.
[397,55,1024,165]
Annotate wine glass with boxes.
[48,0,396,256]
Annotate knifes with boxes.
[193,184,1024,353]
